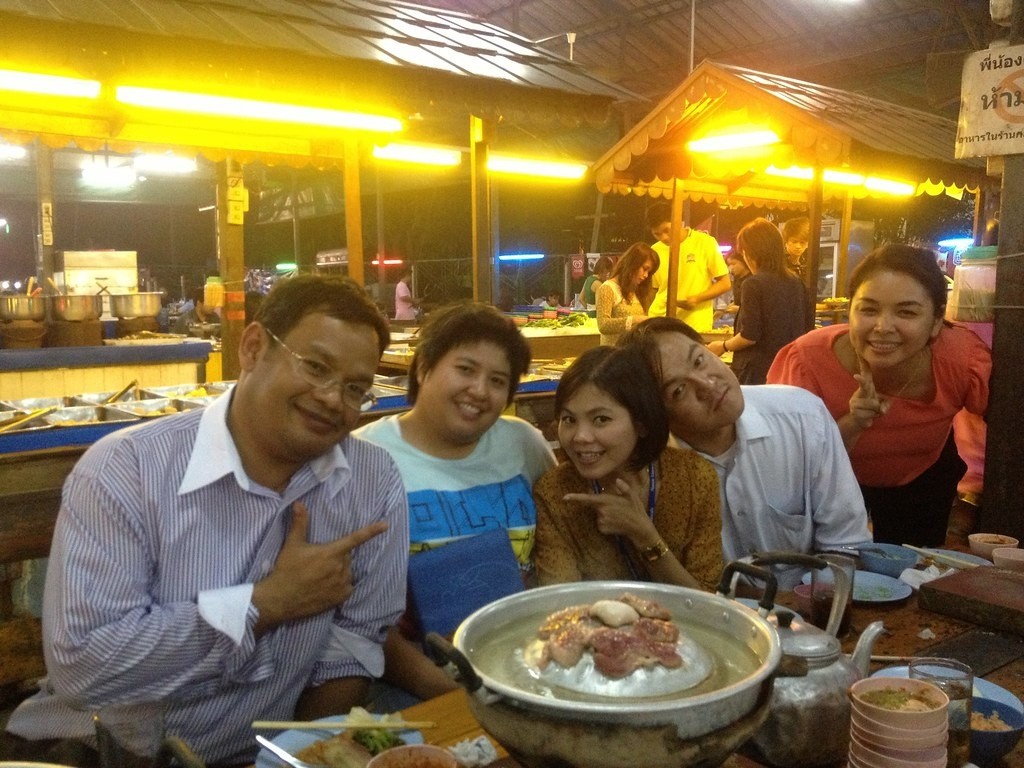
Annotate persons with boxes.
[706,216,815,385]
[646,202,732,333]
[984,221,998,246]
[395,269,424,320]
[596,243,661,345]
[712,274,735,330]
[616,319,873,602]
[0,269,408,768]
[341,298,560,715]
[782,216,809,280]
[534,345,725,592]
[172,287,221,339]
[579,256,614,318]
[765,245,993,548]
[724,249,751,313]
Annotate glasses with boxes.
[265,327,378,413]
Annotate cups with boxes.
[808,553,855,644]
[908,657,974,768]
[366,743,457,768]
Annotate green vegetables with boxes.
[352,727,406,757]
[524,311,589,329]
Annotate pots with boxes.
[426,560,782,768]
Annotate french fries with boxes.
[823,297,850,302]
[704,324,734,361]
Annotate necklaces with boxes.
[895,353,922,396]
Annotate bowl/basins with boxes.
[857,542,918,578]
[109,291,164,318]
[992,547,1024,571]
[822,300,850,308]
[968,533,1019,561]
[794,582,851,636]
[971,696,1024,768]
[0,294,46,321]
[50,295,103,322]
[847,676,949,768]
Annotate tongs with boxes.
[0,405,57,432]
[100,378,137,405]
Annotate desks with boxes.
[515,317,601,359]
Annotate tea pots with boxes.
[713,548,888,768]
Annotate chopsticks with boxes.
[251,719,439,730]
[901,543,980,567]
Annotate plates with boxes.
[916,548,993,570]
[801,568,912,603]
[255,713,424,768]
[870,666,1024,715]
[503,303,569,325]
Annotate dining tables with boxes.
[396,545,1024,768]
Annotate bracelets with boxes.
[723,340,728,352]
[640,538,669,562]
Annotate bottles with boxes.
[202,276,225,309]
